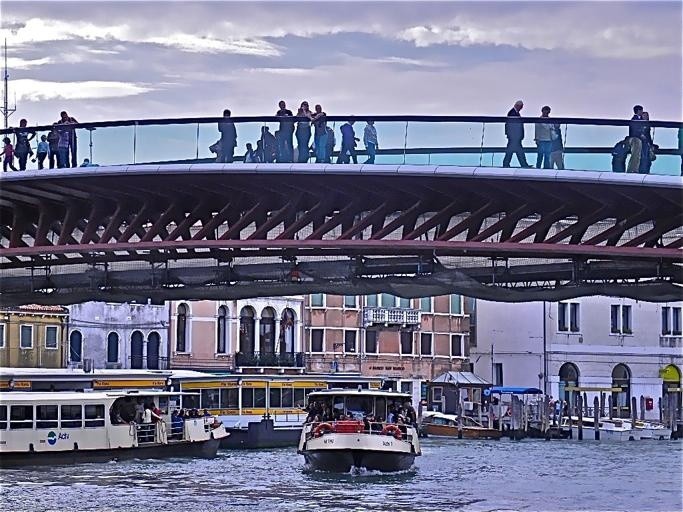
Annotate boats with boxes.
[421,415,672,441]
[295,386,423,473]
[0,377,331,470]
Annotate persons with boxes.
[0,110,84,171]
[109,399,210,441]
[209,100,379,165]
[501,100,565,171]
[611,106,658,173]
[678,126,683,176]
[297,398,417,439]
[491,397,498,406]
[545,394,568,416]
